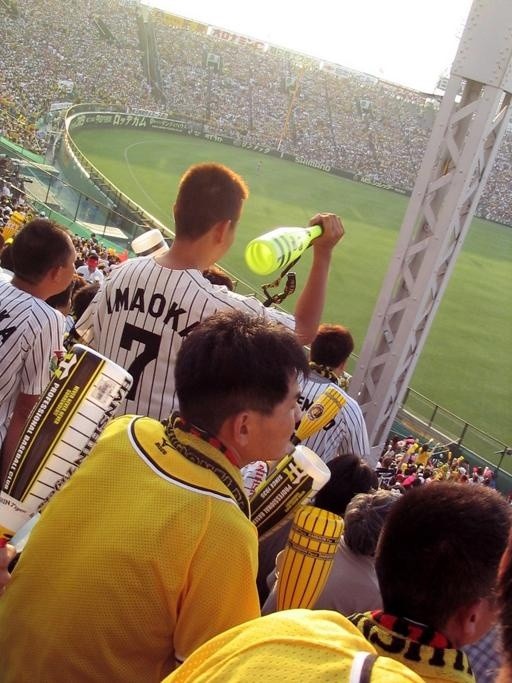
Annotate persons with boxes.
[0,218,76,492]
[74,161,344,423]
[258,455,379,607]
[378,433,498,487]
[261,488,403,620]
[0,309,311,683]
[162,481,511,683]
[2,0,512,330]
[297,324,371,464]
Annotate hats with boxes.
[130,228,173,258]
